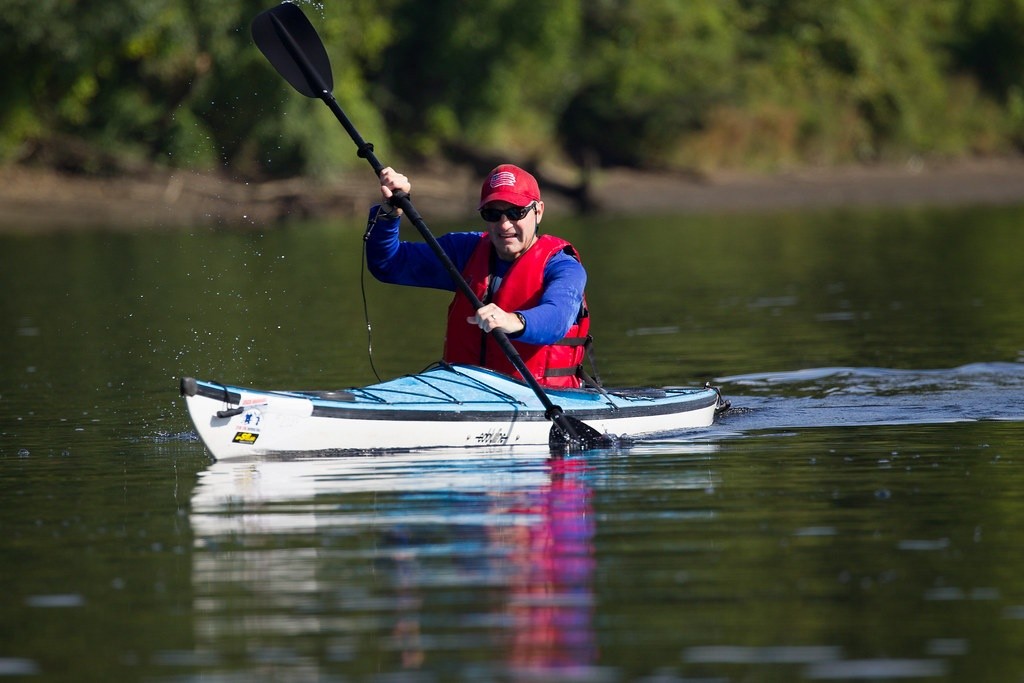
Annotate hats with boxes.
[477,163,540,210]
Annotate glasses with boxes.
[479,202,536,223]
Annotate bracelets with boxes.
[515,313,526,327]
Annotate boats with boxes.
[180,363,732,461]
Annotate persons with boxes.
[365,164,590,387]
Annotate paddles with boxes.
[249,2,611,450]
